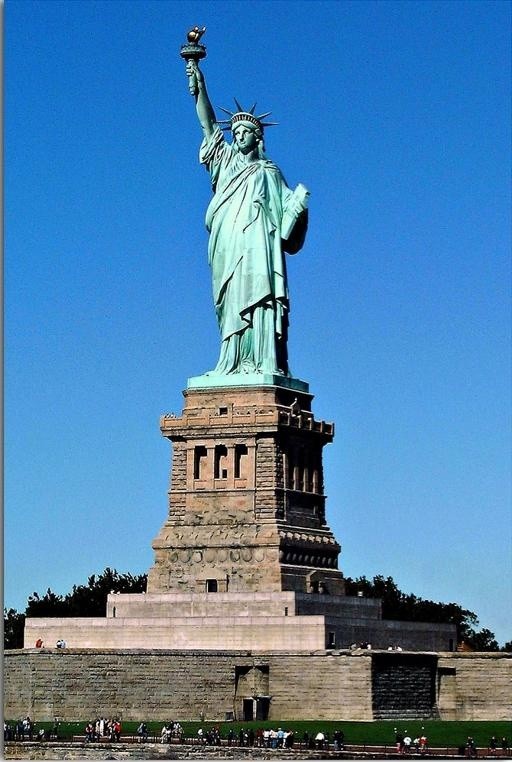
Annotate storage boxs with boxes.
[33,731,329,749]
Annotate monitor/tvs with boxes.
[281,184,311,240]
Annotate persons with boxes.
[393,734,429,753]
[4,718,59,742]
[136,721,343,750]
[35,638,43,648]
[186,62,311,376]
[466,737,508,756]
[388,642,403,652]
[350,640,372,650]
[56,639,65,649]
[84,716,121,744]
